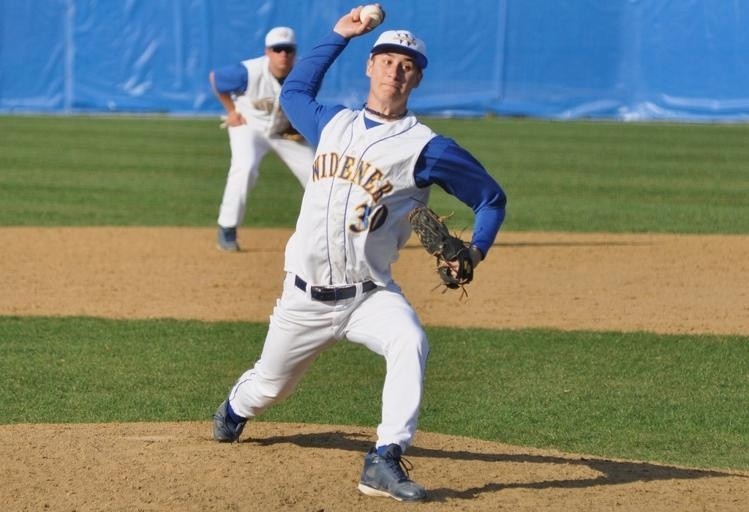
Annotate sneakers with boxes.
[210,392,249,444]
[216,224,241,254]
[359,443,425,503]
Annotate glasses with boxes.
[270,45,294,54]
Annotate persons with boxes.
[210,26,316,254]
[210,3,508,504]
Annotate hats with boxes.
[370,27,428,70]
[265,26,299,47]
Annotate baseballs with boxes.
[360,5,382,27]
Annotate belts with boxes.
[292,272,379,302]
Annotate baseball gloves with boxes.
[408,207,474,289]
[281,122,303,141]
